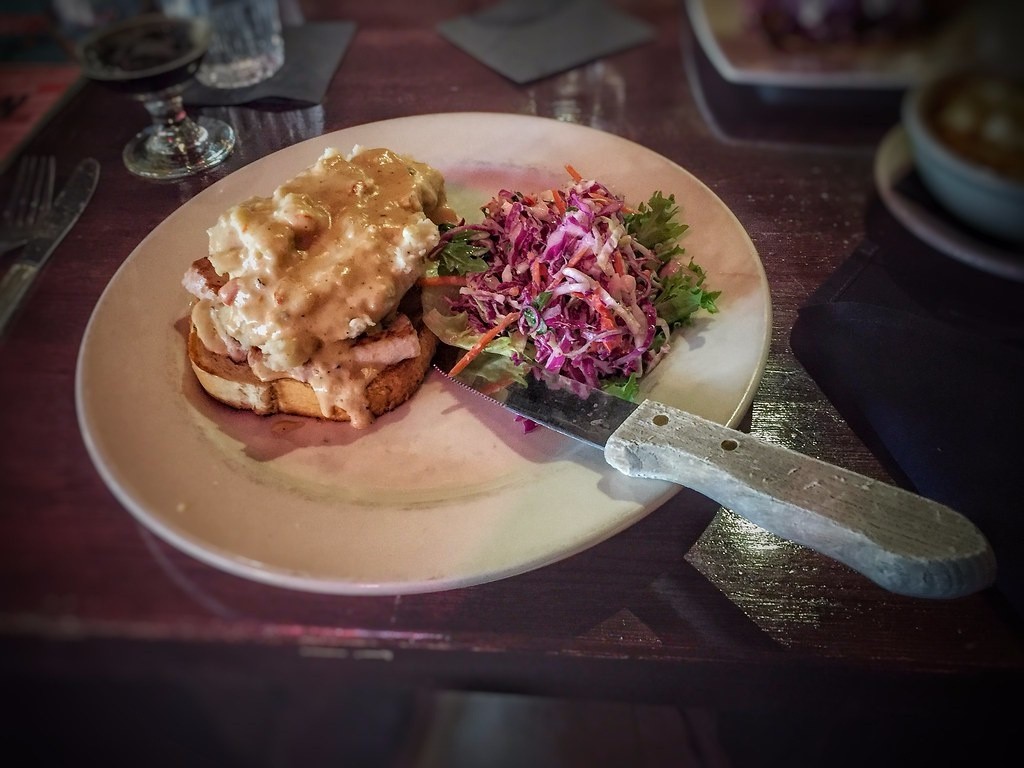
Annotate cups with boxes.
[162,0,284,90]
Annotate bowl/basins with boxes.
[905,78,1024,236]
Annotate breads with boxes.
[189,310,437,423]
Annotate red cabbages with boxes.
[425,177,670,434]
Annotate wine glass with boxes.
[55,0,234,179]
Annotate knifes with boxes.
[0,159,100,332]
[434,346,987,599]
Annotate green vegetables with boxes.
[437,190,725,405]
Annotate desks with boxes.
[0,0,1024,714]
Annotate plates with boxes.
[74,113,774,595]
[686,0,953,88]
[874,126,1024,279]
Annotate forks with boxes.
[0,158,56,257]
